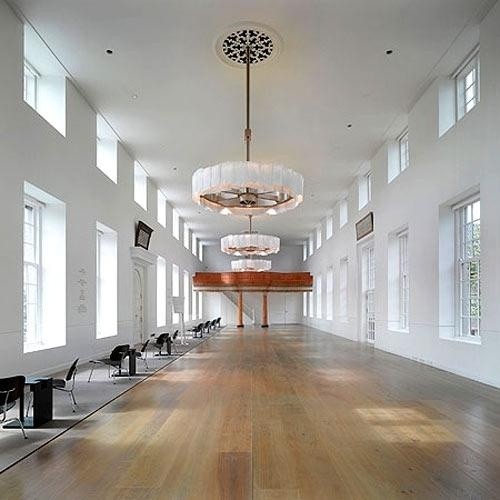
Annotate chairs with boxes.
[87,315,222,386]
[0,358,80,439]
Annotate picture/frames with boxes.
[134,220,155,249]
[355,213,374,240]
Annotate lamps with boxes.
[191,20,305,274]
